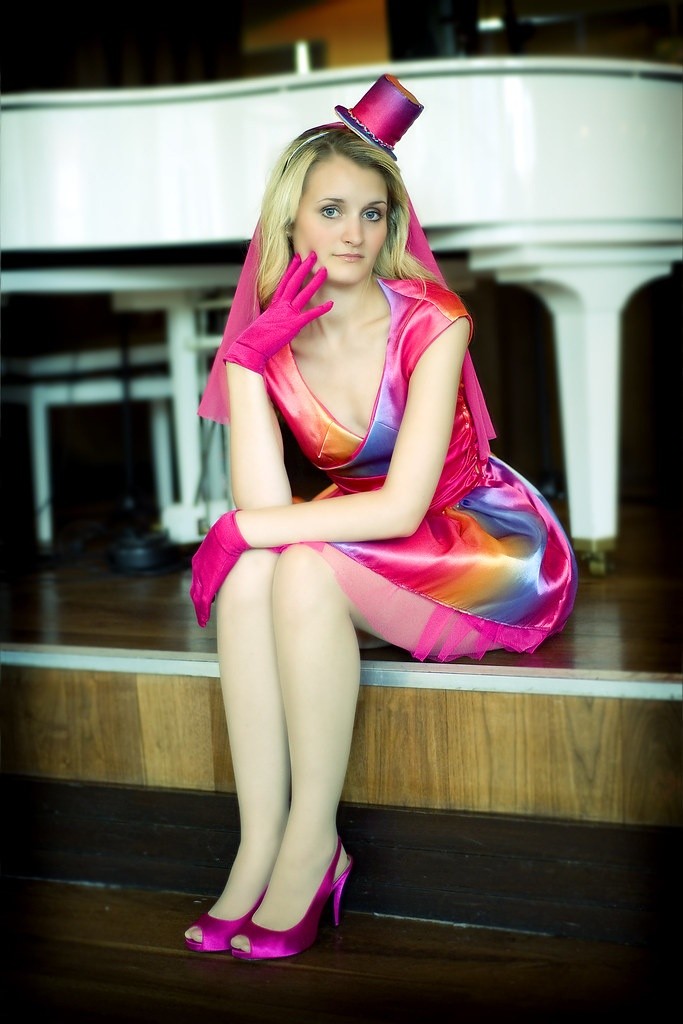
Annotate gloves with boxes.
[222,251,334,375]
[190,508,255,626]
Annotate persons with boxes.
[184,70,580,960]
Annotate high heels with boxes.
[185,883,269,952]
[231,837,355,961]
